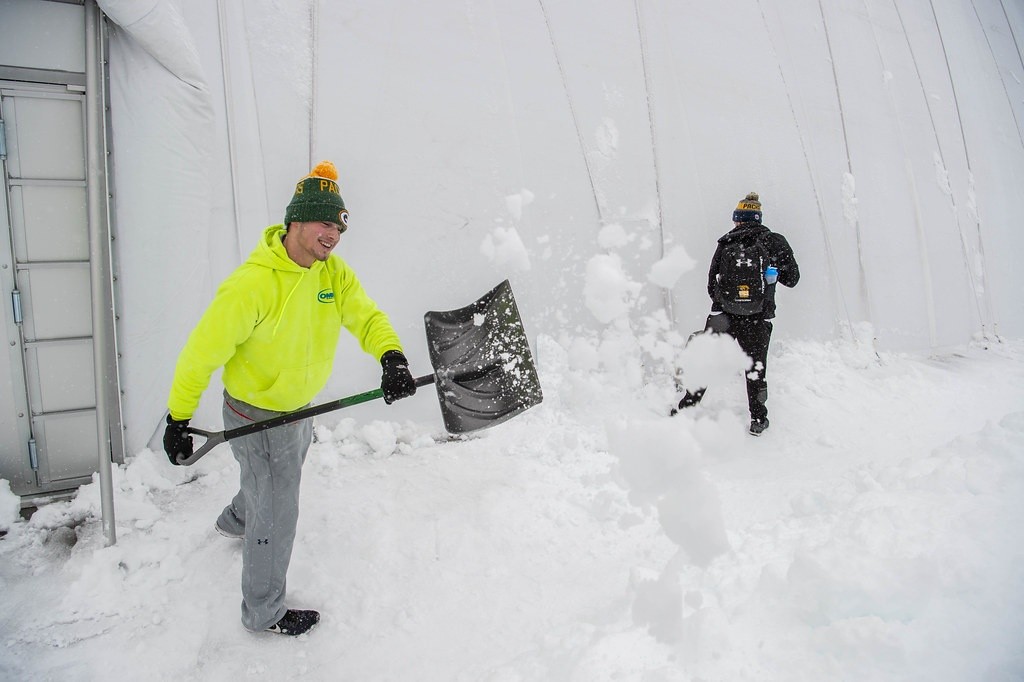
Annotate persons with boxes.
[666,193,802,434]
[164,162,419,638]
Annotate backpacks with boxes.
[714,231,777,316]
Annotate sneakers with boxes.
[750,418,770,436]
[241,609,321,638]
[214,521,245,539]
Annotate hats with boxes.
[732,192,762,224]
[284,161,349,233]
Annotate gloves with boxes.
[163,414,193,466]
[380,351,416,405]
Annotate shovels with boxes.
[174,278,543,465]
